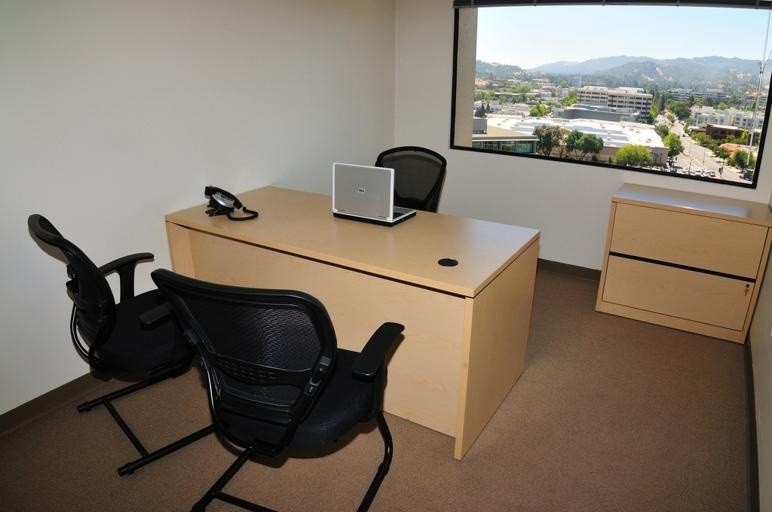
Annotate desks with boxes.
[163,184,542,461]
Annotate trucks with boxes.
[740,167,755,181]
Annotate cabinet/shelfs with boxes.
[593,181,772,346]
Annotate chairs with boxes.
[149,266,405,512]
[27,213,219,478]
[374,145,447,213]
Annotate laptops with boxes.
[332,163,416,228]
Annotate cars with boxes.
[629,156,716,178]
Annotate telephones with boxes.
[205,186,243,216]
